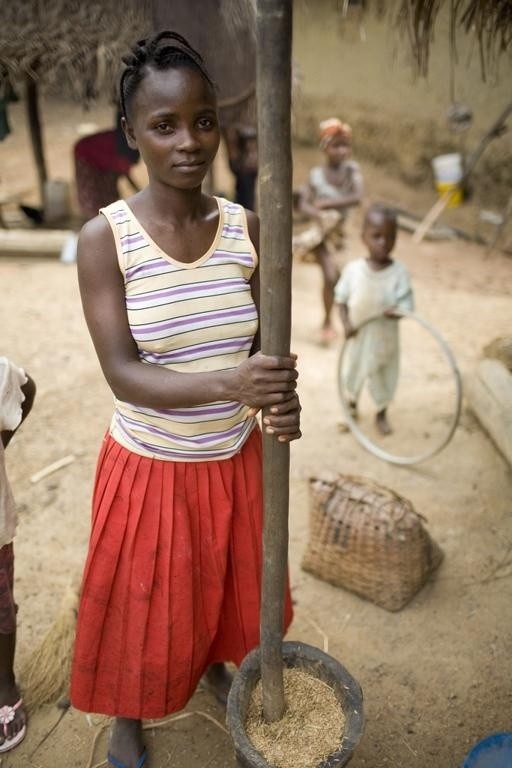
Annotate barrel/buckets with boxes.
[435,182,462,212]
[432,152,463,185]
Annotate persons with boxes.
[326,204,417,439]
[64,28,306,766]
[70,119,137,232]
[0,354,37,754]
[298,117,368,345]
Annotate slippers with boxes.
[2,699,28,752]
[107,750,148,767]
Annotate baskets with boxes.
[301,472,445,612]
